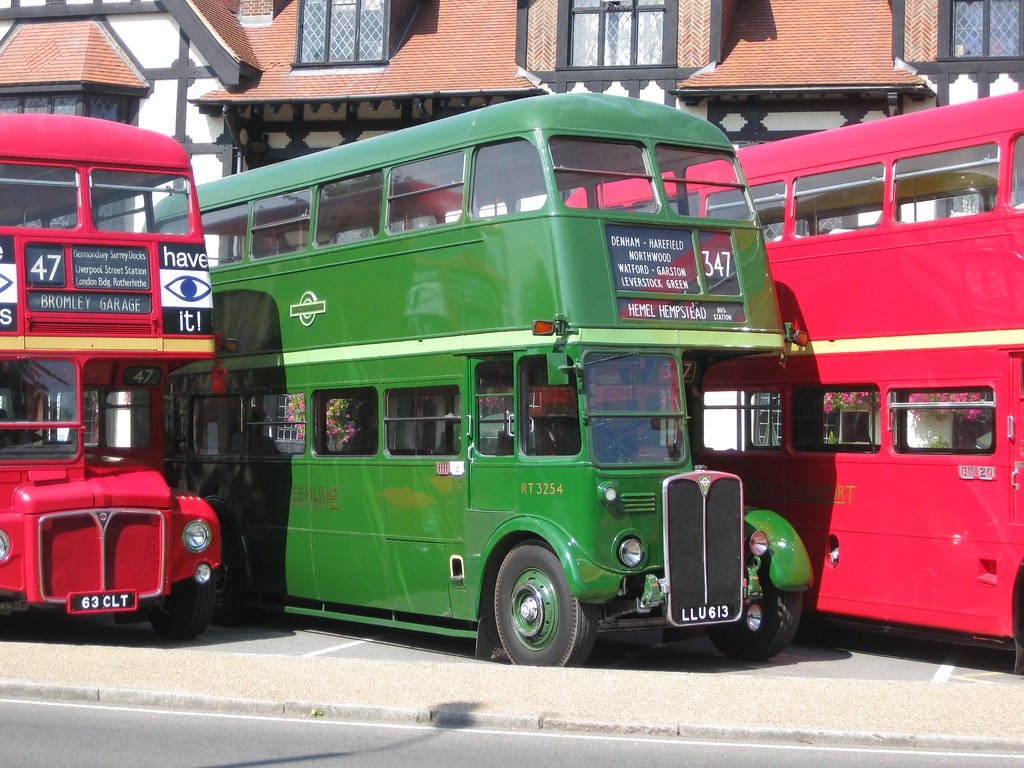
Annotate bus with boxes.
[0,112,223,611]
[192,93,813,668]
[565,89,1024,683]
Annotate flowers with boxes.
[286,393,363,452]
[821,391,880,447]
[908,392,988,449]
